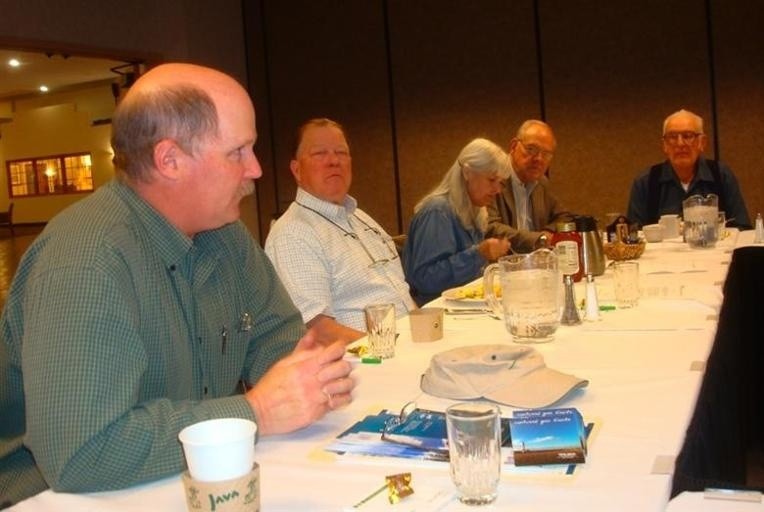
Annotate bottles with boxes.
[583,272,600,321]
[752,213,764,242]
[559,275,581,325]
[551,222,582,282]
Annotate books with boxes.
[324,408,595,476]
[508,407,589,467]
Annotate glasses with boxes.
[295,198,399,268]
[517,139,553,160]
[663,132,706,142]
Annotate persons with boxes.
[0,63,355,509]
[399,137,510,308]
[623,109,755,231]
[484,120,566,254]
[263,118,418,350]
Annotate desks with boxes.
[736,230,764,248]
[0,228,740,512]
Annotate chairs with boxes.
[0,203,14,233]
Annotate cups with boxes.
[614,262,639,308]
[176,417,263,512]
[643,224,663,242]
[716,211,727,239]
[606,213,620,224]
[483,248,563,343]
[660,215,678,238]
[683,194,719,249]
[365,304,396,359]
[409,307,443,343]
[446,403,501,506]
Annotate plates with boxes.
[442,284,503,303]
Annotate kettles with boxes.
[574,216,605,278]
